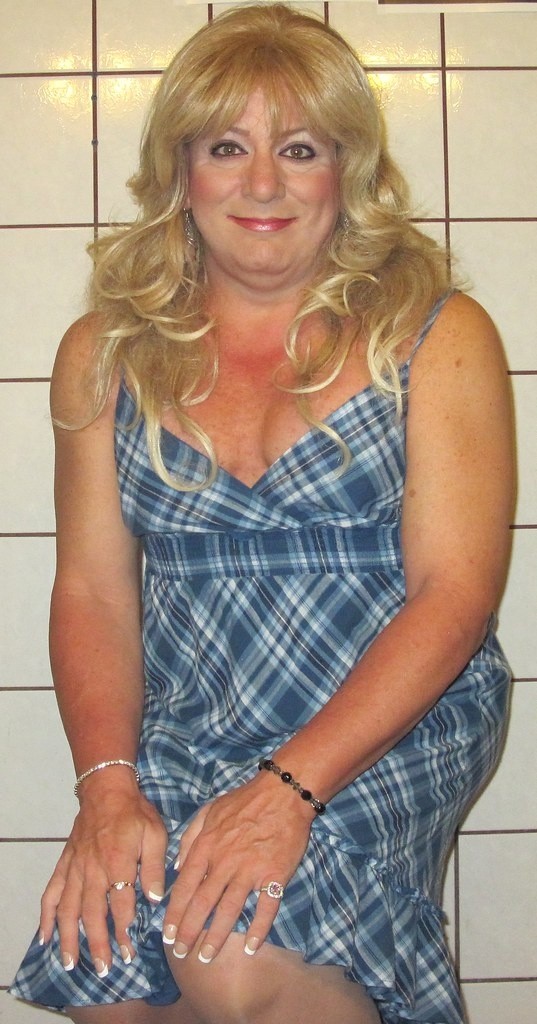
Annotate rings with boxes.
[106,881,136,892]
[259,881,286,900]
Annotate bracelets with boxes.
[257,753,327,817]
[73,759,142,798]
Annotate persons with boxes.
[9,2,515,1024]
